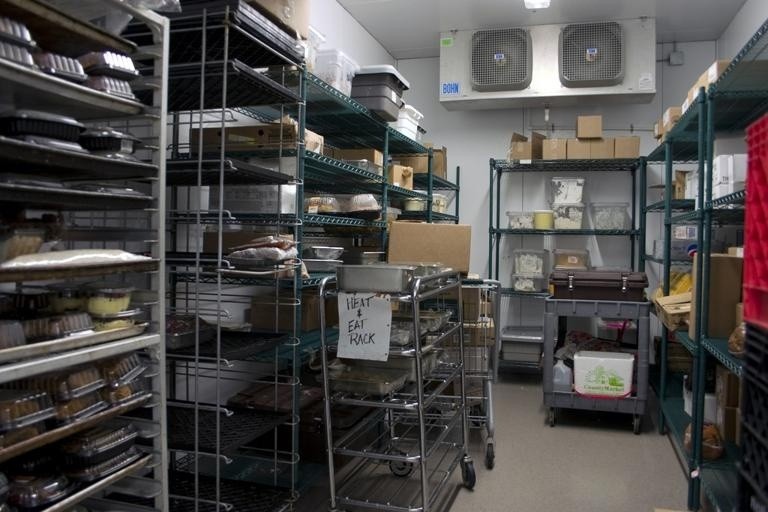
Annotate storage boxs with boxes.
[251,289,338,331]
[416,125,427,144]
[550,177,585,203]
[536,212,553,228]
[506,211,535,226]
[653,239,702,260]
[690,254,742,341]
[513,276,543,292]
[673,226,699,238]
[511,132,546,159]
[614,136,639,158]
[191,124,297,154]
[680,98,690,113]
[340,150,383,176]
[388,103,424,141]
[685,171,696,198]
[728,246,744,255]
[483,301,492,317]
[543,139,566,159]
[255,0,310,41]
[388,165,414,190]
[663,107,681,129]
[392,147,447,179]
[688,59,768,104]
[717,371,739,445]
[567,139,590,159]
[351,65,410,121]
[306,25,326,73]
[654,123,664,138]
[315,49,358,98]
[683,374,716,421]
[502,325,557,363]
[592,202,629,230]
[577,115,602,139]
[728,154,746,205]
[514,249,547,273]
[553,248,588,267]
[654,337,694,373]
[591,139,614,158]
[711,223,745,247]
[675,169,686,198]
[442,318,497,346]
[202,231,295,280]
[574,350,634,398]
[712,154,729,209]
[655,294,690,329]
[387,222,471,273]
[436,285,481,321]
[552,204,586,228]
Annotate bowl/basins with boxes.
[79,282,136,313]
[47,283,83,312]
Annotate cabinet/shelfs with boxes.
[391,166,460,224]
[387,278,502,470]
[544,298,649,434]
[488,161,647,381]
[66,0,306,510]
[0,1,167,512]
[639,87,706,511]
[230,68,433,512]
[319,270,476,511]
[693,18,768,512]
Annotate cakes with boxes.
[0,354,154,447]
[1,284,143,375]
